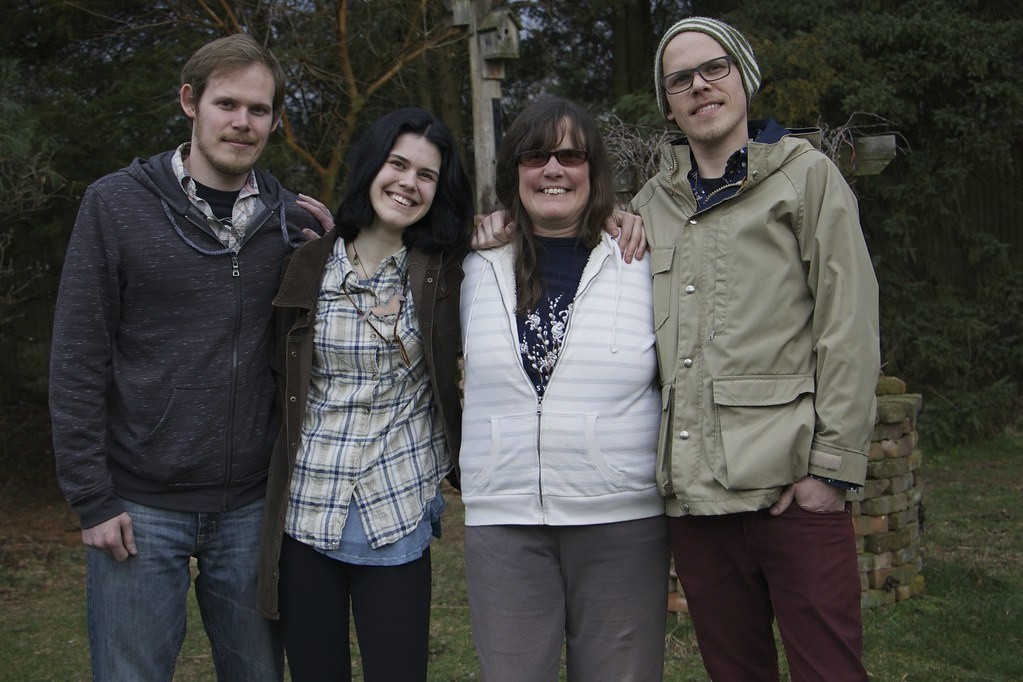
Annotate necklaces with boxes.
[341,239,412,382]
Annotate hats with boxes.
[653,16,761,126]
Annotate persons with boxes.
[471,16,877,682]
[275,111,647,682]
[50,34,323,682]
[460,100,663,682]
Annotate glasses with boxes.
[660,54,737,94]
[517,149,591,168]
